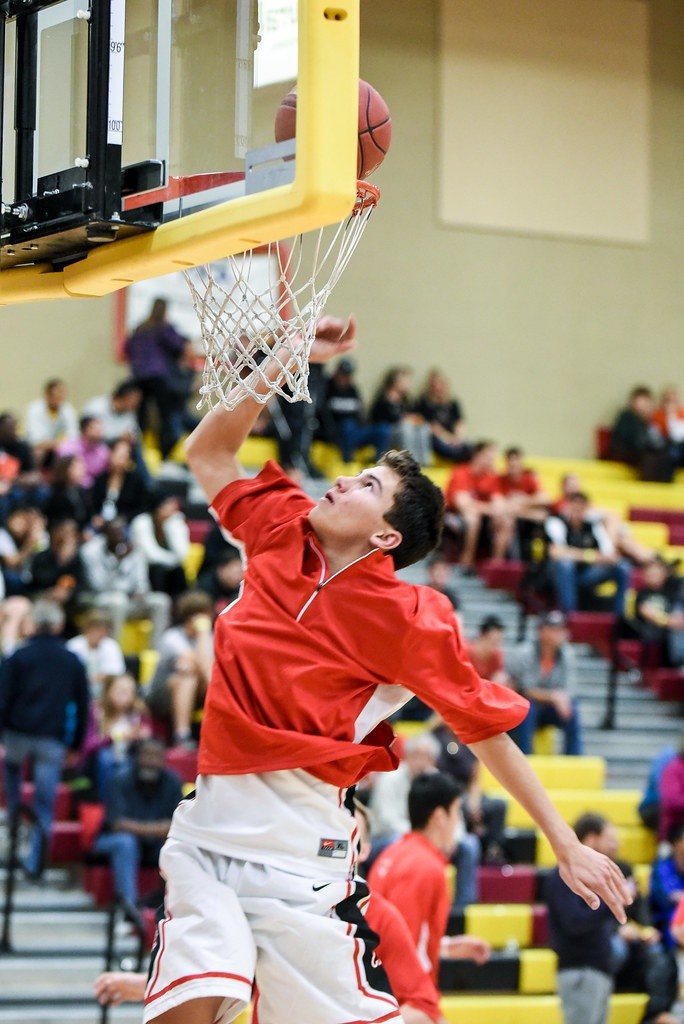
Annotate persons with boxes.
[607,387,675,482]
[228,333,478,476]
[648,385,684,468]
[440,438,684,668]
[0,337,244,937]
[136,315,635,1024]
[358,556,684,1023]
[122,299,183,448]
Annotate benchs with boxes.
[0,725,684,1024]
[141,433,684,703]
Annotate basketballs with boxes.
[272,76,394,182]
